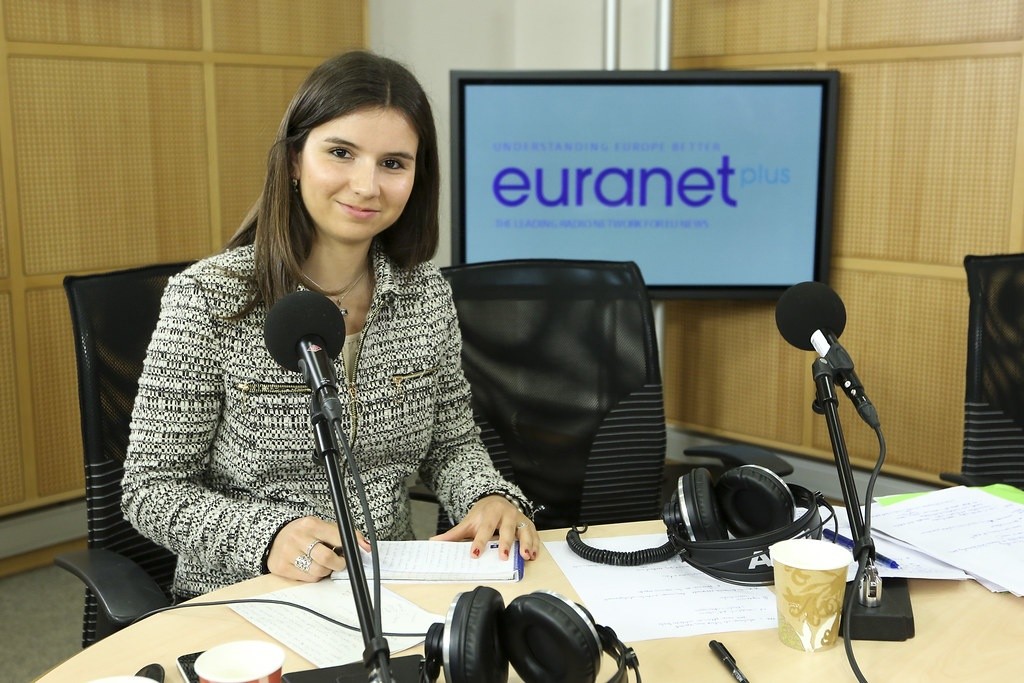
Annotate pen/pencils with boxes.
[821,528,901,571]
[709,640,748,683]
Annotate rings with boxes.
[295,556,313,572]
[517,523,527,530]
[307,540,322,557]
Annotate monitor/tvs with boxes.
[449,69,839,302]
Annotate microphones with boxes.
[264,292,347,422]
[775,282,881,428]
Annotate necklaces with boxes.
[302,272,366,315]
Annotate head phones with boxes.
[422,585,643,683]
[662,463,839,587]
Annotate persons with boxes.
[120,51,538,606]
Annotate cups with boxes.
[769,539,854,653]
[89,675,159,683]
[193,640,286,683]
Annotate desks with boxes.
[32,508,1024,683]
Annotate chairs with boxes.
[437,259,788,535]
[52,261,199,647]
[939,253,1024,492]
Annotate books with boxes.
[873,483,1024,592]
[330,540,524,584]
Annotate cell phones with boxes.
[177,651,207,683]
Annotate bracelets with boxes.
[487,490,523,513]
